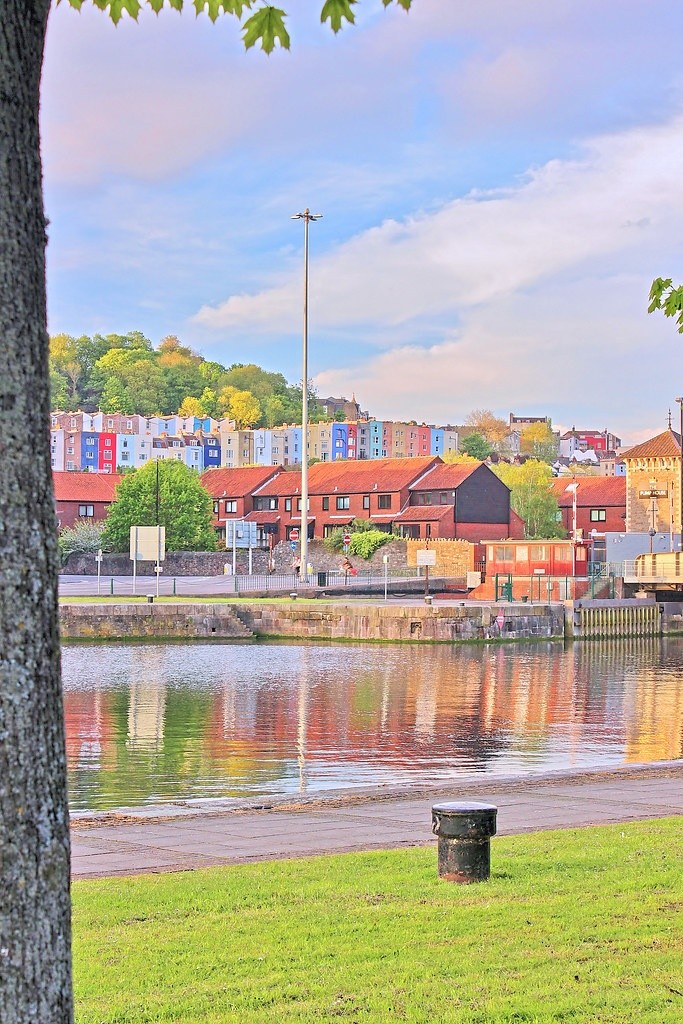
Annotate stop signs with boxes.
[289,530,298,541]
[343,535,351,545]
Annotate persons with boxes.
[339,556,355,576]
[289,556,301,577]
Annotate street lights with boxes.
[289,207,323,584]
[674,395,683,551]
[562,481,579,577]
